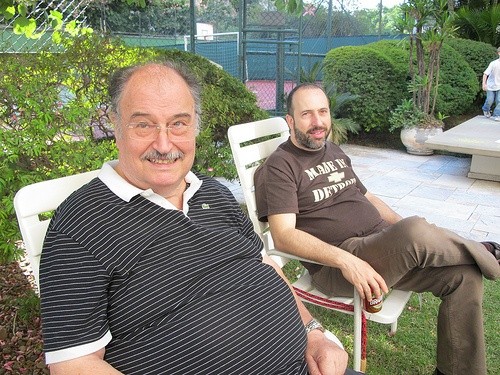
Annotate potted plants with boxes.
[401,1,462,156]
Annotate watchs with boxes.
[304,317,325,334]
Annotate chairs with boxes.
[15,171,345,354]
[227,118,411,371]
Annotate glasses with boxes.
[115,108,197,142]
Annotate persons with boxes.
[39,62,367,375]
[253,81,500,375]
[481,46,500,120]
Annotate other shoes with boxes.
[482,109,491,118]
[493,115,500,121]
